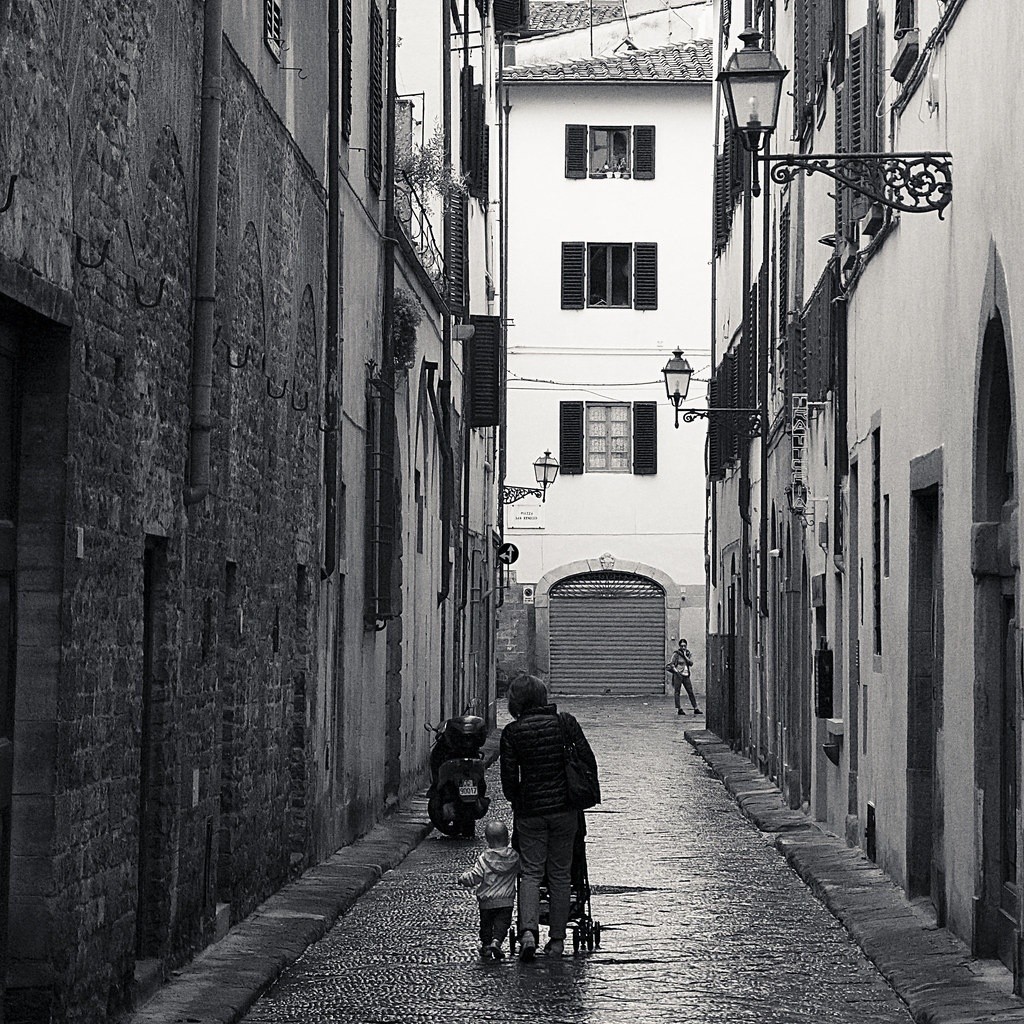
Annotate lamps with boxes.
[532,449,562,503]
[715,21,790,198]
[661,346,694,429]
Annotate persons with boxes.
[459,821,523,960]
[501,675,597,964]
[670,639,703,714]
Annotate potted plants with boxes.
[602,158,631,179]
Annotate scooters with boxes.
[425,698,490,838]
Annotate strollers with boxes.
[506,801,601,952]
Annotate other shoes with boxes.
[479,944,491,957]
[520,930,536,960]
[489,941,505,959]
[678,709,686,715]
[694,709,703,715]
[548,939,564,960]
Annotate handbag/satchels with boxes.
[560,713,597,808]
